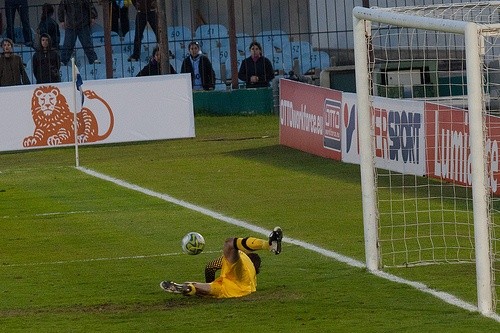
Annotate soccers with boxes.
[182,232,205,255]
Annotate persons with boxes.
[100,0,130,42]
[128,0,175,62]
[181,42,216,91]
[238,42,275,89]
[38,2,60,50]
[159,227,283,301]
[135,48,177,77]
[32,33,62,84]
[0,38,31,87]
[58,0,101,66]
[5,0,33,47]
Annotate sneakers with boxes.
[268,226,283,255]
[160,280,192,294]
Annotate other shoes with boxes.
[128,58,138,62]
[92,60,102,64]
[168,50,175,60]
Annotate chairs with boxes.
[0,19,332,91]
